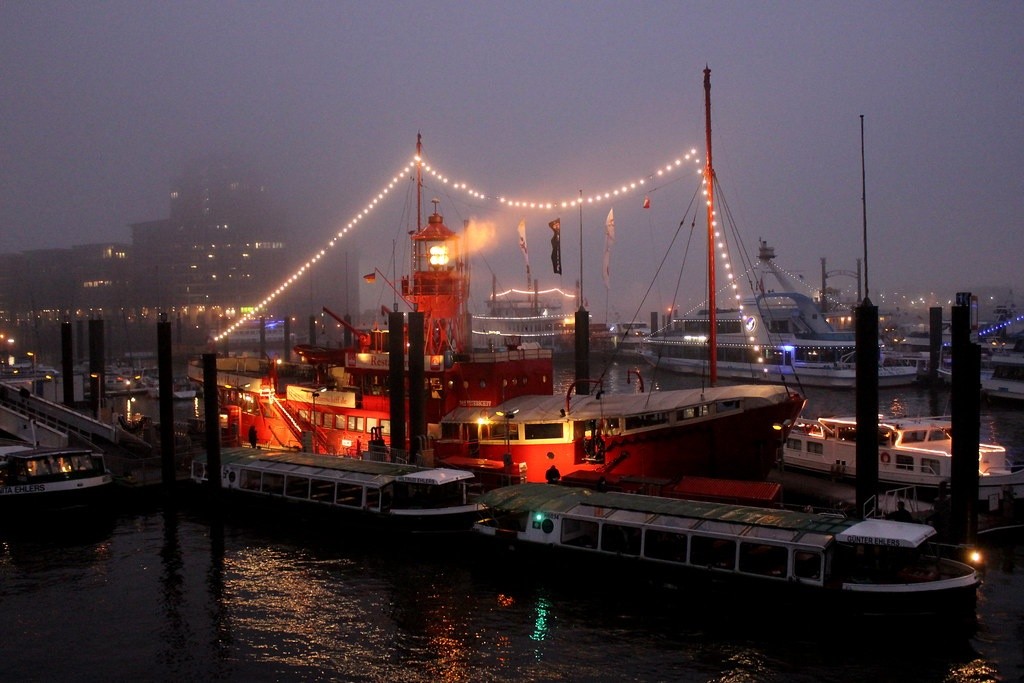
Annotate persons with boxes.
[883,502,913,523]
[546,465,560,484]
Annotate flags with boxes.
[549,218,562,275]
[644,198,650,208]
[517,218,532,291]
[363,273,375,283]
[602,209,614,290]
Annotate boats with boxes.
[638,288,919,391]
[475,482,979,620]
[615,321,651,361]
[105,363,196,404]
[184,62,802,506]
[0,443,133,539]
[939,344,1023,400]
[206,320,307,349]
[781,415,1006,495]
[192,445,479,535]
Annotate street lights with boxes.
[46,375,57,404]
[26,352,37,394]
[92,372,101,421]
[117,375,141,422]
